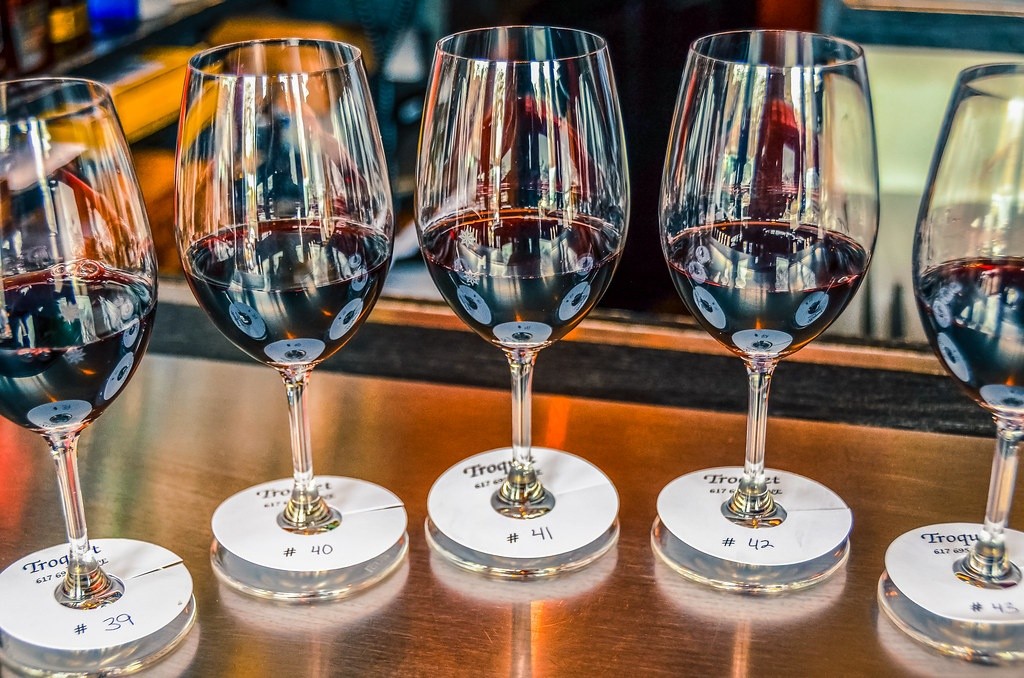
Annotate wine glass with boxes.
[877,61,1024,665]
[413,25,630,580]
[0,77,199,678]
[650,28,881,596]
[173,37,409,601]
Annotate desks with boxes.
[1,355,1024,677]
